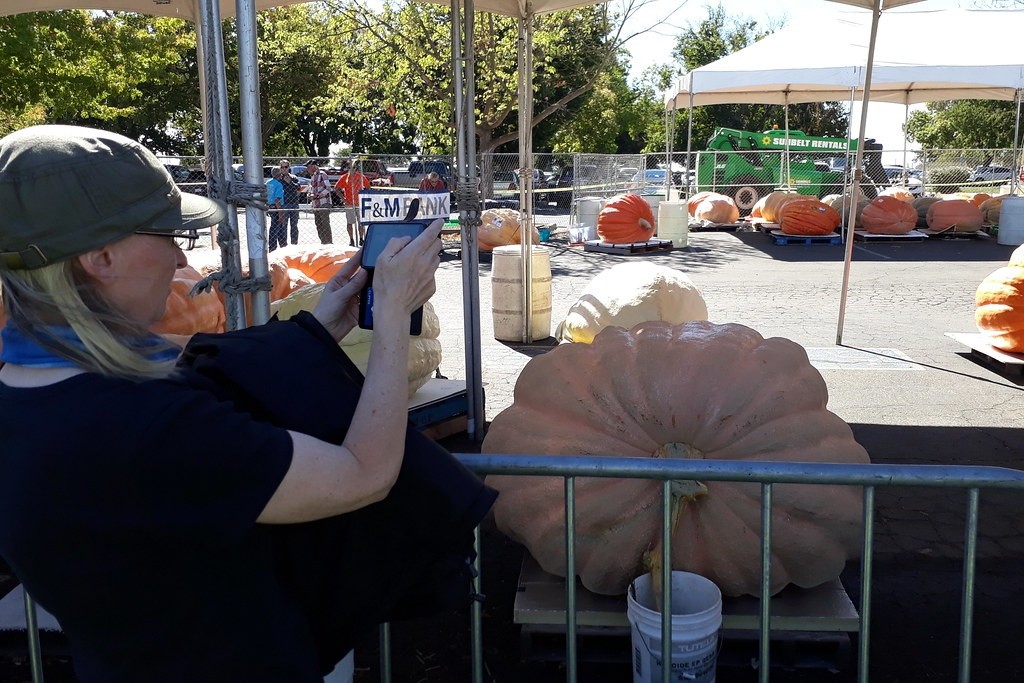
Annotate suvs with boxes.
[356,159,390,188]
[965,165,1014,186]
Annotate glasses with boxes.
[281,166,288,169]
[134,227,199,251]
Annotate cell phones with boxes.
[357,281,424,336]
[360,223,426,270]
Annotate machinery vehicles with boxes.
[694,127,890,217]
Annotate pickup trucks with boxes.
[392,161,457,190]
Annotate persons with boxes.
[0,124,448,683]
[418,172,445,253]
[263,165,287,253]
[333,161,371,247]
[279,159,301,248]
[302,159,333,245]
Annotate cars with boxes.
[513,168,548,202]
[883,165,922,196]
[628,170,675,196]
[615,168,638,188]
[163,164,352,207]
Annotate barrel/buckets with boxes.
[538,228,551,242]
[998,198,1024,246]
[576,196,602,242]
[626,572,722,683]
[490,244,551,342]
[640,194,665,235]
[657,201,688,248]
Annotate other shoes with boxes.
[349,240,365,247]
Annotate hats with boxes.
[303,159,319,166]
[0,123,227,271]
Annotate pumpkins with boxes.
[143,243,440,402]
[480,321,872,598]
[974,244,1024,353]
[687,187,1005,235]
[597,194,656,245]
[475,208,540,252]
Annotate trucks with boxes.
[548,165,602,208]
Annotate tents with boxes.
[0,1,928,347]
[662,6,1024,201]
